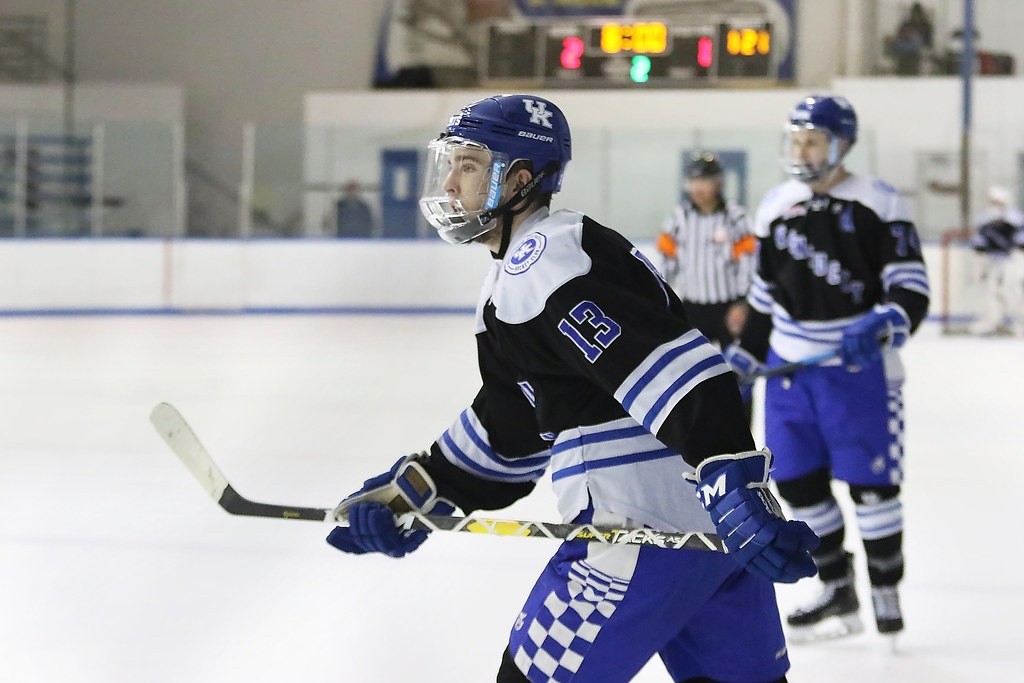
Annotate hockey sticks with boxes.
[737,333,892,387]
[145,397,728,557]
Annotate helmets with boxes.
[781,95,858,182]
[419,95,573,247]
[684,153,723,179]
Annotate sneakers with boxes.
[787,552,865,643]
[871,585,903,652]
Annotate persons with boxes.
[933,28,963,74]
[325,93,821,683]
[969,187,1024,336]
[659,151,756,428]
[337,181,373,238]
[896,4,933,75]
[725,94,931,654]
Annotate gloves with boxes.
[841,303,909,375]
[325,450,456,558]
[682,447,821,583]
[724,346,761,399]
